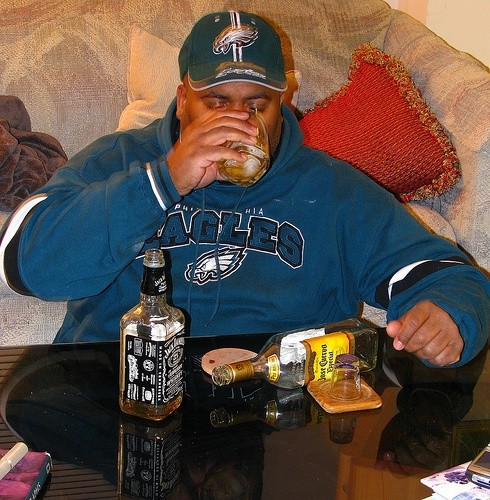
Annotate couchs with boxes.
[0,1,490,345]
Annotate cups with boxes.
[216,112,270,186]
[329,354,361,401]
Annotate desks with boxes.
[0,326,490,500]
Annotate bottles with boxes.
[118,248,186,420]
[212,317,379,389]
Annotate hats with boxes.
[178,12,288,92]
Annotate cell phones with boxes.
[465,442,490,488]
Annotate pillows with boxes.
[114,27,180,140]
[291,45,462,201]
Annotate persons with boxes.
[0,9,490,370]
[0,343,488,500]
